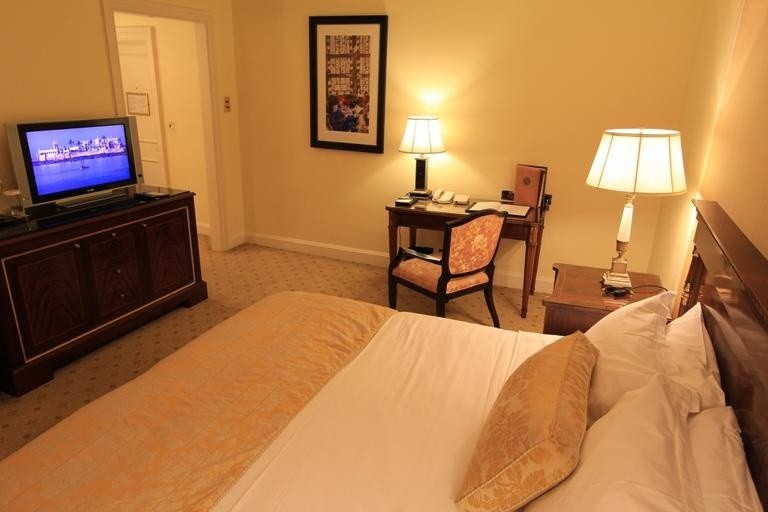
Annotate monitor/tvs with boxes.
[4,113,145,216]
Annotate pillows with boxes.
[583,291,680,432]
[686,404,764,512]
[523,371,700,512]
[454,329,600,512]
[665,301,729,421]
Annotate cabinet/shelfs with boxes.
[0,183,209,399]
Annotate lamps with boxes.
[584,127,688,288]
[397,115,449,200]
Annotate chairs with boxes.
[387,207,509,328]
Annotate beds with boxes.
[0,199,768,512]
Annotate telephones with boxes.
[432,187,454,204]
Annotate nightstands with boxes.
[541,262,666,335]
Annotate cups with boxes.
[4,190,26,219]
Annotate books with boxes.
[465,201,531,218]
[513,164,548,223]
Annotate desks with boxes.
[384,192,547,318]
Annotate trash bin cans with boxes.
[408,247,434,255]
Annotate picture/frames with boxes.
[308,15,389,155]
[125,92,150,116]
